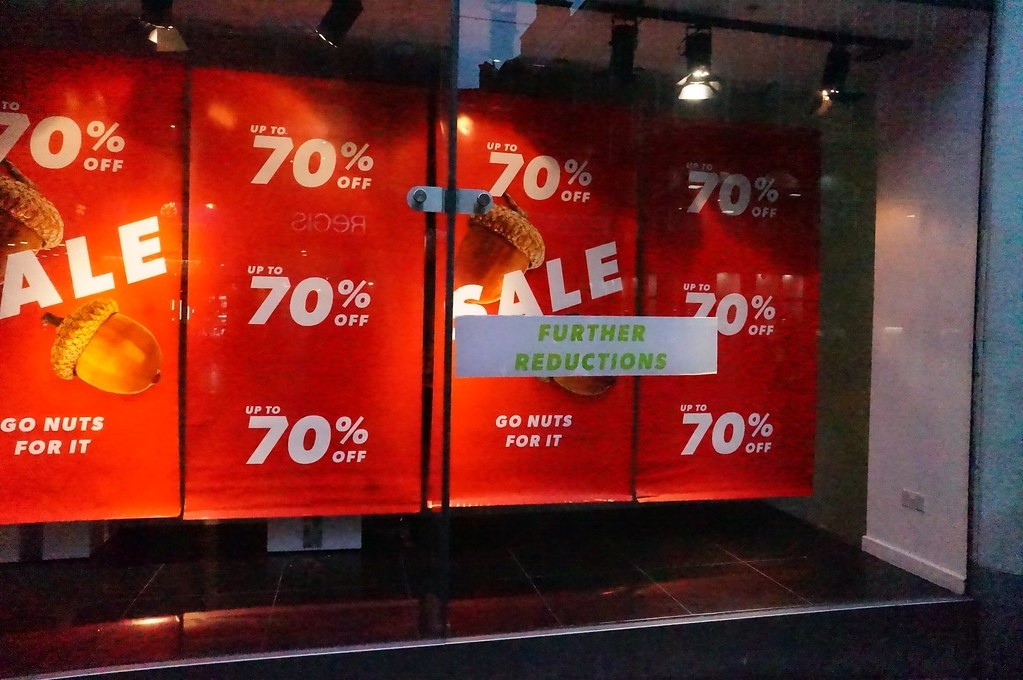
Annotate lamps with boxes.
[821,47,852,99]
[608,19,643,86]
[139,0,173,30]
[316,0,363,48]
[675,27,722,101]
